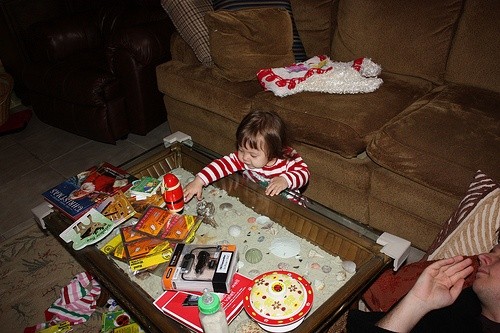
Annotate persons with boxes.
[355,226,500,333]
[69,162,133,201]
[181,111,310,209]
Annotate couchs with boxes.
[0,0,174,145]
[157,0,500,251]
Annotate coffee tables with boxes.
[30,131,411,333]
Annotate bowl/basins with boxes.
[244,271,313,333]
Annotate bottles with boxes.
[197,287,228,333]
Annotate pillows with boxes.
[203,7,295,81]
[211,0,308,64]
[420,168,500,261]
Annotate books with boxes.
[42,161,139,221]
